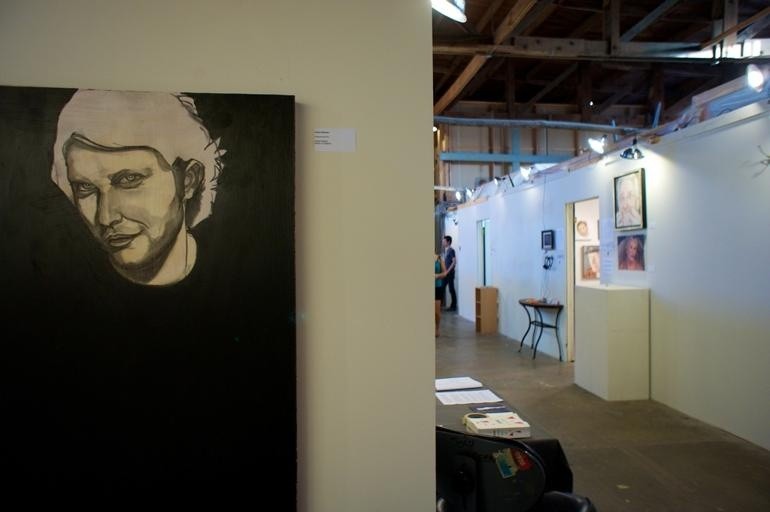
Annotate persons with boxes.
[583,246,599,280]
[435,248,448,337]
[616,173,640,228]
[619,235,644,269]
[441,237,457,311]
[0,90,295,512]
[576,221,595,239]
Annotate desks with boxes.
[518,297,564,362]
[436,375,555,443]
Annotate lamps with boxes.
[455,191,466,202]
[466,186,477,199]
[432,0,468,23]
[518,165,535,182]
[587,134,608,155]
[493,176,506,186]
[620,132,644,160]
[746,63,770,93]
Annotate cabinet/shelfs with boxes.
[475,287,498,336]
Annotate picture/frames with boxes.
[542,230,554,250]
[612,168,645,231]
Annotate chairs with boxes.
[436,426,596,512]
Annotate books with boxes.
[466,412,532,439]
